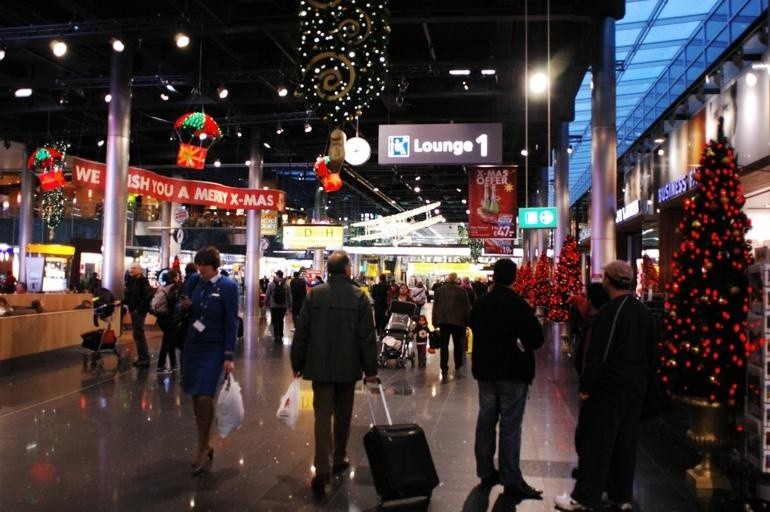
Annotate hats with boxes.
[274,270,283,278]
[601,261,634,285]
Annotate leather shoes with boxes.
[332,461,350,474]
[481,468,499,486]
[132,360,149,367]
[505,478,543,498]
[312,473,329,486]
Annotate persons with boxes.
[290,249,383,490]
[261,270,323,343]
[154,367,178,411]
[571,281,610,480]
[80,272,100,292]
[470,259,545,498]
[357,272,493,382]
[115,264,199,369]
[173,244,239,477]
[128,370,149,416]
[0,269,45,317]
[552,260,660,510]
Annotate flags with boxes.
[467,165,518,240]
[483,238,514,254]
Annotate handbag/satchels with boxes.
[174,318,192,350]
[429,329,440,349]
[80,329,116,351]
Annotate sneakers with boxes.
[156,367,173,374]
[601,492,633,511]
[554,493,592,511]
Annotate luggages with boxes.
[362,376,439,512]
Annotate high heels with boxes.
[191,446,214,467]
[190,463,208,478]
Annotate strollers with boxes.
[379,301,419,370]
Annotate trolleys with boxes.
[80,299,123,360]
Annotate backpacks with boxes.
[93,289,115,326]
[151,284,175,313]
[271,280,286,305]
